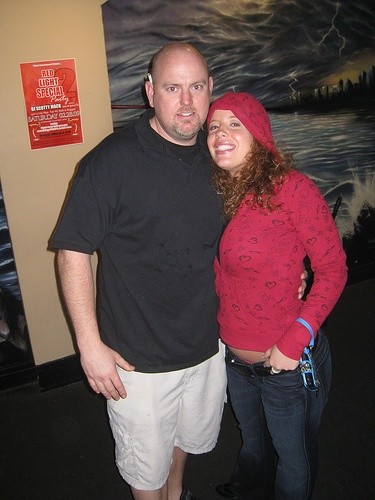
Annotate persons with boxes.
[204,93,349,500]
[46,42,228,500]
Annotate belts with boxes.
[225,345,294,377]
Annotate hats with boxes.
[208,92,280,159]
